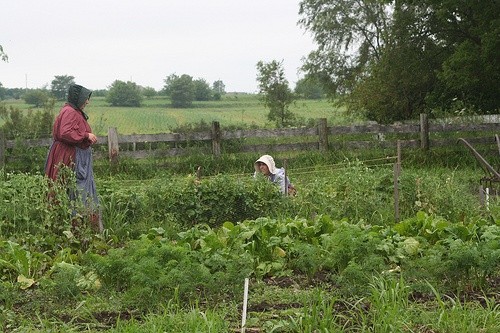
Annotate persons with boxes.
[253,155,297,198]
[44,84,103,239]
